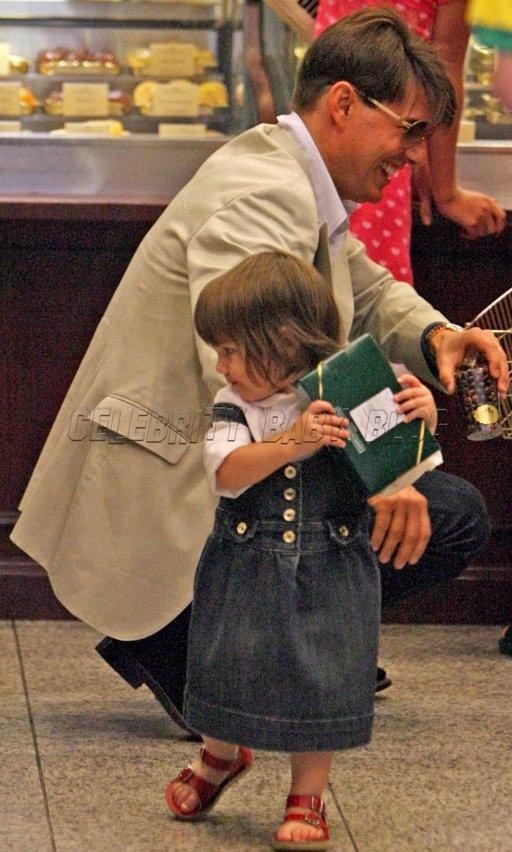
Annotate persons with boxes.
[9,5,512,742]
[310,0,506,291]
[161,248,440,852]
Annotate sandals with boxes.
[274,793,332,844]
[166,745,256,818]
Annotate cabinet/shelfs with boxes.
[1,1,511,200]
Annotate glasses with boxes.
[352,86,428,138]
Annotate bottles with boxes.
[452,365,502,443]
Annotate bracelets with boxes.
[423,322,464,360]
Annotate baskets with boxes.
[461,283,512,439]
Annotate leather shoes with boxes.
[95,625,202,736]
